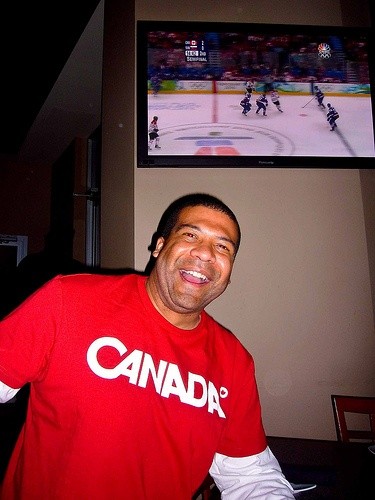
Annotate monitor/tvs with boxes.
[136,19,375,169]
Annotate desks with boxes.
[207,436,375,500]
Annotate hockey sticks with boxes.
[302,96,315,108]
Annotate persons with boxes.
[149,115,161,149]
[7,224,88,427]
[151,73,162,96]
[245,78,256,96]
[327,103,340,131]
[0,194,301,500]
[240,94,251,116]
[147,31,370,83]
[313,86,325,109]
[263,73,275,94]
[256,93,268,115]
[271,89,284,112]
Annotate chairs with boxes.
[331,395,375,442]
